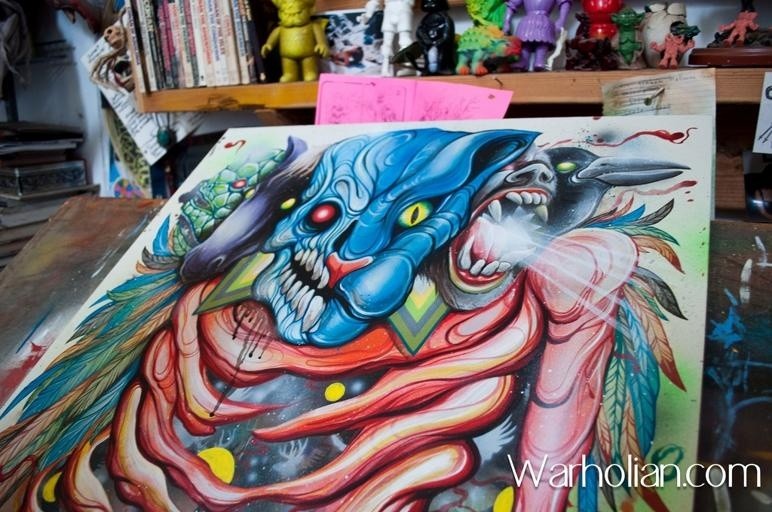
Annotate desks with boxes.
[0,194,772,512]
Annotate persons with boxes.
[258,0,761,84]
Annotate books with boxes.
[0,118,100,275]
[122,0,270,95]
